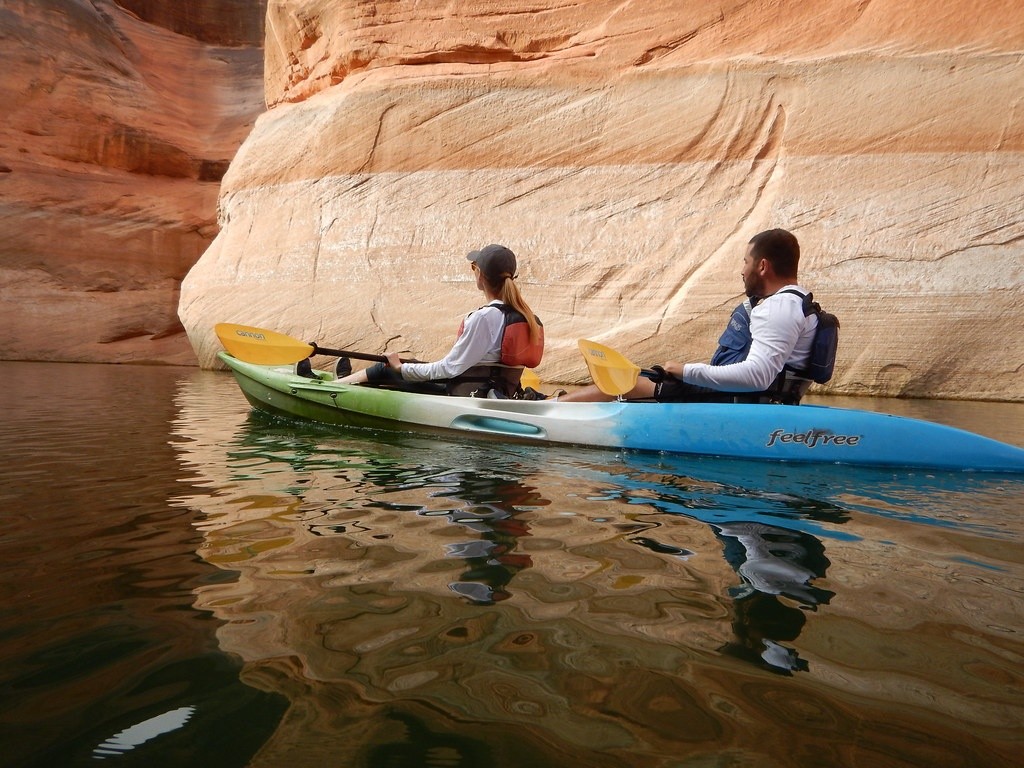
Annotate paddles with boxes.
[213,321,541,393]
[575,336,677,398]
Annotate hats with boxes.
[467,244,516,281]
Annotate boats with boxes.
[216,348,1024,478]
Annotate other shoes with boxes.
[487,388,511,399]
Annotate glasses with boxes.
[470,261,477,271]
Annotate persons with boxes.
[296,243,539,396]
[487,227,822,405]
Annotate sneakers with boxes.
[293,357,323,380]
[333,356,352,381]
